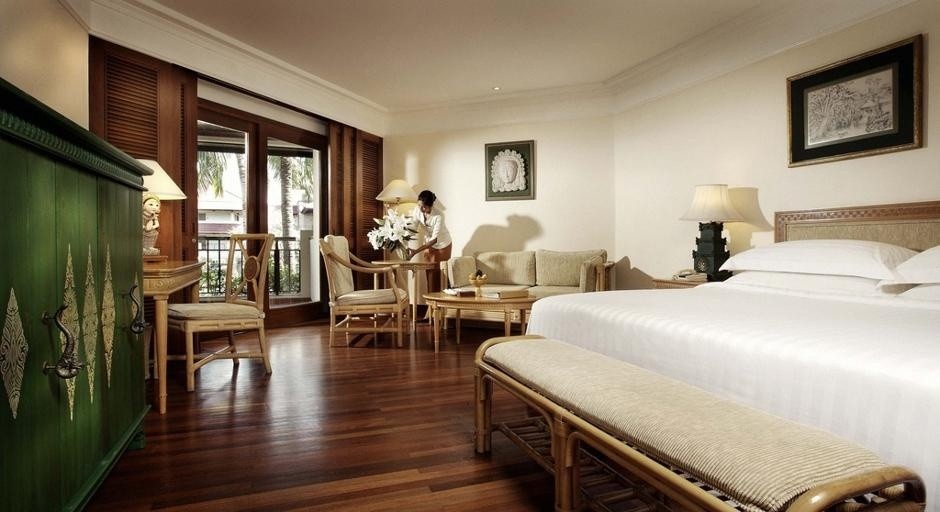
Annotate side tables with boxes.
[371,260,438,335]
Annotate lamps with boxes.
[375,180,418,216]
[678,184,744,282]
[135,159,188,257]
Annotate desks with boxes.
[144,261,206,415]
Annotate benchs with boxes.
[474,334,926,512]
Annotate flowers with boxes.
[366,209,419,253]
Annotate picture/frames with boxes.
[786,34,923,168]
[485,140,535,201]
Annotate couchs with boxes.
[440,249,615,330]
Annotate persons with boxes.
[143,194,160,255]
[405,190,452,324]
[491,149,526,193]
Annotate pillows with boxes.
[536,250,599,286]
[579,256,601,291]
[447,256,476,289]
[719,240,939,286]
[474,251,535,286]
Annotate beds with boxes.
[526,200,940,510]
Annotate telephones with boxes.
[672,269,696,280]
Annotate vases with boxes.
[394,245,410,260]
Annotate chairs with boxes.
[318,235,410,348]
[167,234,275,392]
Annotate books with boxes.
[480,289,528,299]
